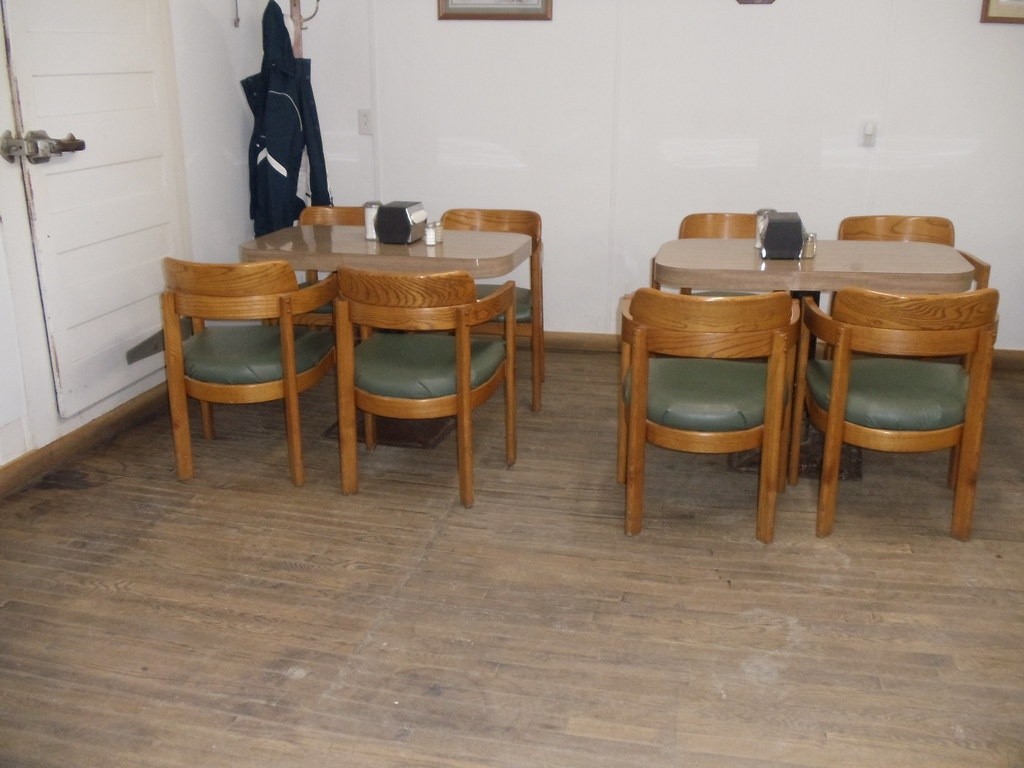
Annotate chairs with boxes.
[160,256,338,487]
[439,208,544,412]
[837,216,991,291]
[334,265,517,509]
[617,288,799,544]
[260,206,366,330]
[651,212,761,297]
[789,287,999,542]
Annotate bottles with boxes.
[754,208,771,248]
[802,233,816,259]
[363,201,382,240]
[425,223,436,245]
[432,221,443,243]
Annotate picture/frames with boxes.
[980,0,1024,24]
[437,0,553,21]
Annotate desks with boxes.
[238,224,532,450]
[654,238,974,482]
